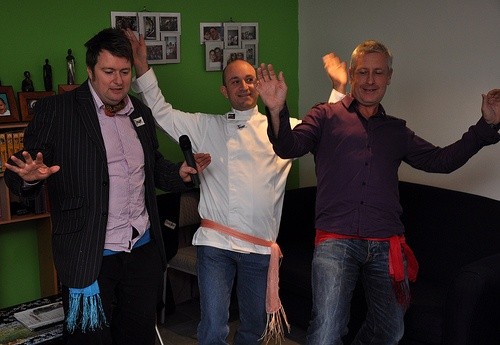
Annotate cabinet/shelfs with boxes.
[0,125,65,345]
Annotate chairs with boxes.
[159,187,200,326]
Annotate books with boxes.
[0,130,29,173]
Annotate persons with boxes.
[65,48,76,85]
[21,70,34,92]
[121,28,349,345]
[42,59,53,92]
[204,27,256,68]
[4,27,211,345]
[253,39,500,345]
[0,98,11,116]
[114,16,177,61]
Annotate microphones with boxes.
[179,135,201,187]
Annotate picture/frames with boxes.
[110,12,182,65]
[18,90,56,122]
[0,86,20,122]
[200,22,259,72]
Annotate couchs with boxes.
[276,181,500,345]
